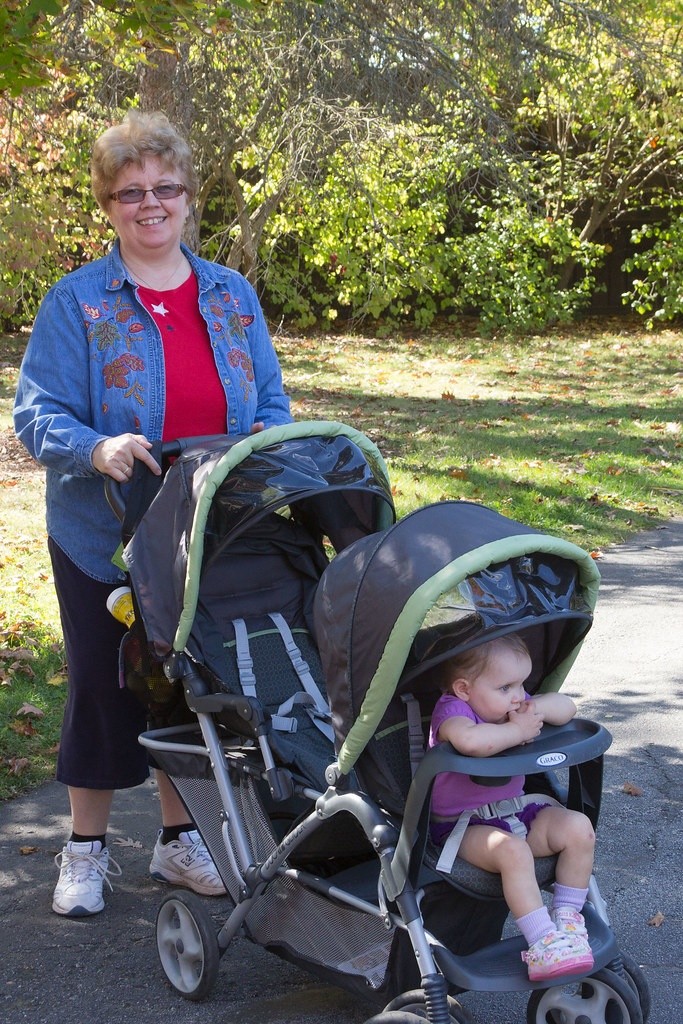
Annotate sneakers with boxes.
[52,840,123,918]
[146,829,231,898]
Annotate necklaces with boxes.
[120,254,183,290]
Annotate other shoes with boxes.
[549,907,591,943]
[522,932,595,981]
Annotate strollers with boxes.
[104,420,654,1023]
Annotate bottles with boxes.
[104,585,135,628]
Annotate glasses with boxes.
[105,185,189,204]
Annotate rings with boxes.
[123,467,130,474]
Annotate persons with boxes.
[13,110,298,918]
[425,633,596,983]
